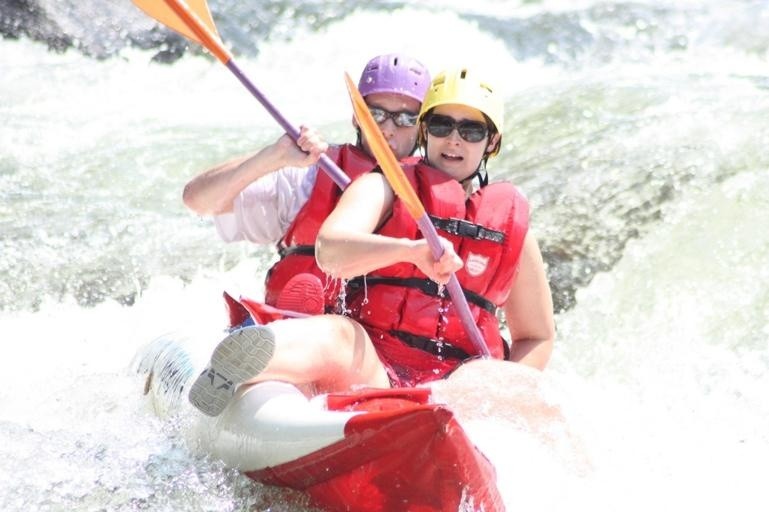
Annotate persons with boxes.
[187,67,554,418]
[181,52,432,385]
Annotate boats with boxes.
[145,326,504,512]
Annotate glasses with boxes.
[367,104,419,128]
[426,114,490,143]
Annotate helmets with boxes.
[356,52,432,104]
[417,67,503,159]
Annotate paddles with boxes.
[132,1,352,189]
[345,73,491,357]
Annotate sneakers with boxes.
[187,324,276,418]
[276,271,325,318]
